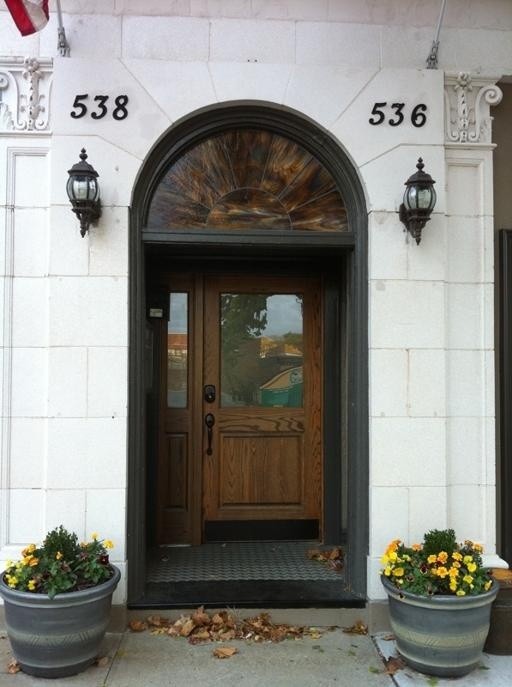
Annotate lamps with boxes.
[398,152,436,246]
[66,144,103,238]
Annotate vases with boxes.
[381,574,498,680]
[1,565,121,678]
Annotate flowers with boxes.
[379,524,494,600]
[2,523,116,599]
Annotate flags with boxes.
[5,0,51,38]
